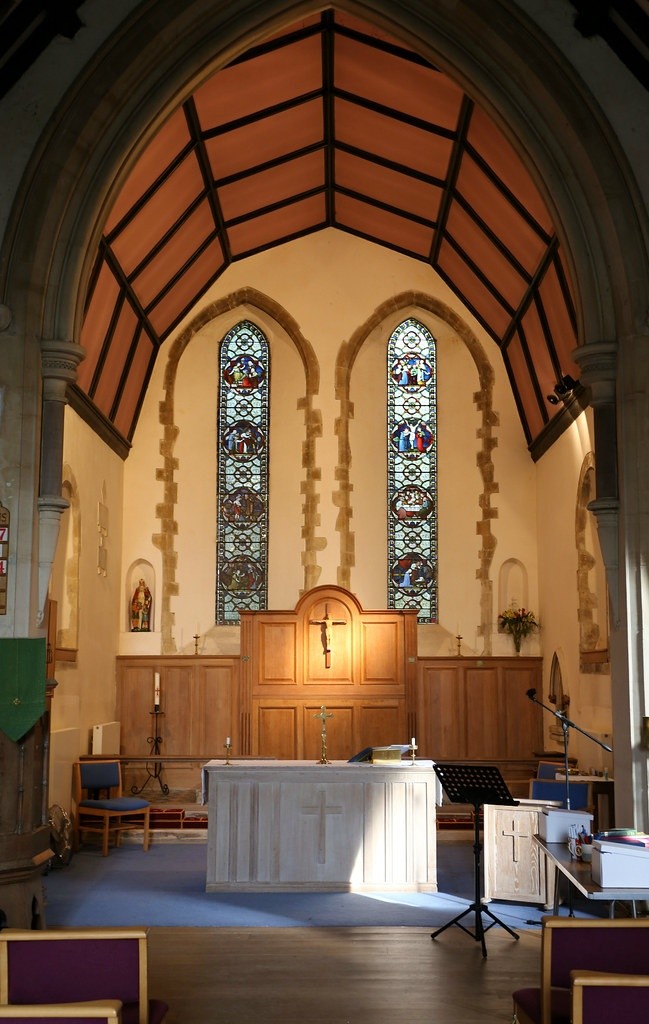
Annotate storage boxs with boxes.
[538,807,595,843]
[591,839,649,889]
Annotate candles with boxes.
[154,672,160,705]
[458,623,461,635]
[195,623,198,635]
[411,737,415,746]
[226,736,230,744]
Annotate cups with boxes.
[566,837,595,862]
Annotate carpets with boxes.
[43,845,611,926]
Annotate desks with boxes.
[555,773,615,833]
[201,758,443,893]
[526,834,649,926]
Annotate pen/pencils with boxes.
[569,824,585,839]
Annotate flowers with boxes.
[498,598,539,656]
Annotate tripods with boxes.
[431,763,521,959]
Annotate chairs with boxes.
[73,759,150,857]
[513,917,649,1024]
[529,761,593,811]
[0,925,170,1024]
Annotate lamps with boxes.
[547,375,581,405]
